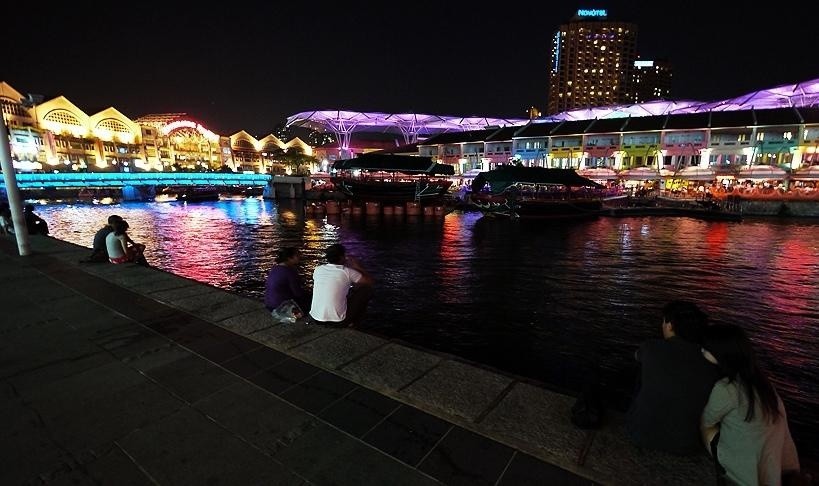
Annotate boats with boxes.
[176,185,221,202]
[470,166,607,221]
[330,154,455,200]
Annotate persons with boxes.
[626,301,800,486]
[265,247,312,312]
[309,244,378,327]
[93,215,146,264]
[0,203,48,236]
